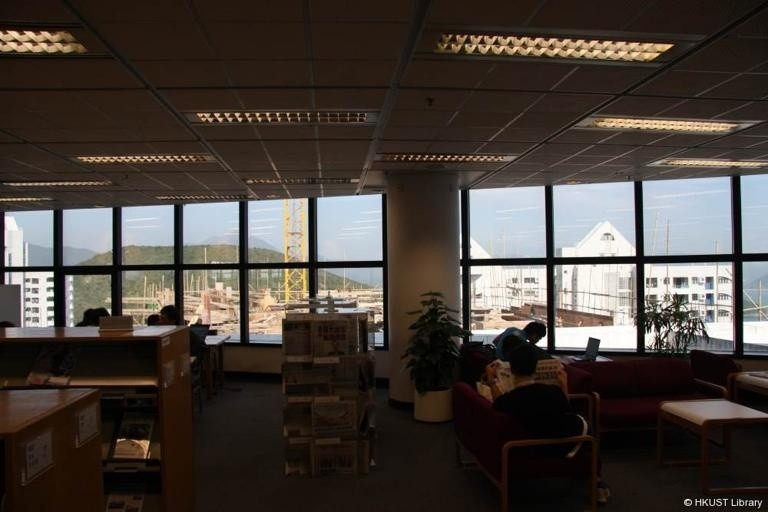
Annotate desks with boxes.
[190,356,196,364]
[205,335,230,400]
[727,370,768,400]
[551,355,614,364]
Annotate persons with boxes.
[492,342,578,478]
[147,305,207,368]
[487,322,569,369]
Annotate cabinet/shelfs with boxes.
[280,296,375,480]
[0,388,104,512]
[0,325,195,512]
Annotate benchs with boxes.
[569,357,728,448]
[454,381,597,512]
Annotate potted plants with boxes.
[397,291,471,423]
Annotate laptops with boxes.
[573,337,600,362]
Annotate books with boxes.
[281,319,358,475]
